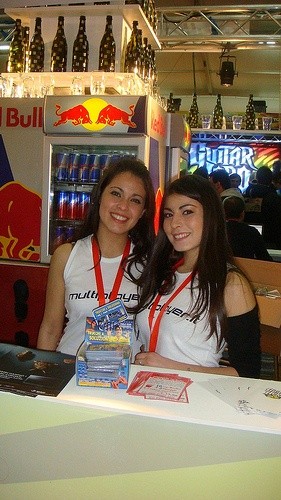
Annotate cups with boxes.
[232,115,244,130]
[262,116,272,130]
[0,72,136,98]
[202,114,213,130]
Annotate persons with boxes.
[107,326,131,343]
[194,167,209,180]
[136,173,262,378]
[229,174,242,193]
[243,160,281,249]
[37,156,156,358]
[209,169,244,216]
[223,196,273,261]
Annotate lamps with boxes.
[215,45,239,87]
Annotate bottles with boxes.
[124,0,158,96]
[98,15,116,73]
[72,15,89,71]
[167,93,256,131]
[25,17,46,72]
[50,15,68,72]
[6,18,29,74]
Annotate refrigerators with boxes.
[41,96,166,271]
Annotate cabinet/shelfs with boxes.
[1,5,162,97]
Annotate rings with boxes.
[140,362,142,364]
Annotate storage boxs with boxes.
[226,121,246,130]
[225,112,246,121]
[256,113,281,131]
[230,256,281,330]
[75,317,134,389]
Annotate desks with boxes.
[0,366,281,500]
